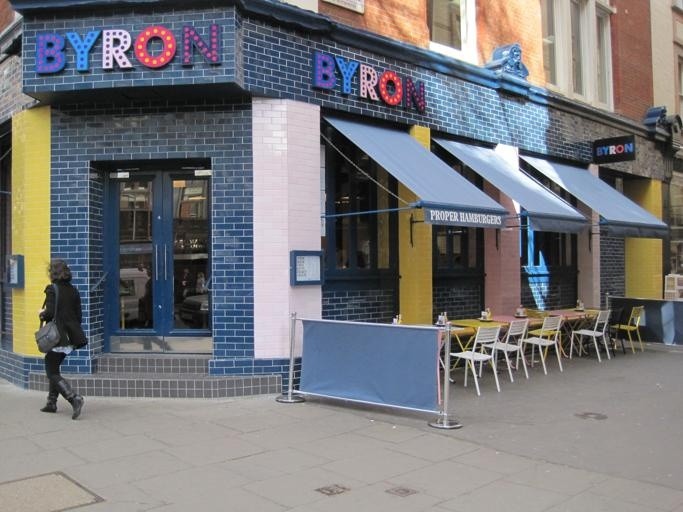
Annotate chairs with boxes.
[448,306,645,397]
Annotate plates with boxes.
[434,323,450,326]
[516,316,528,318]
[481,319,493,322]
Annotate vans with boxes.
[118,267,151,323]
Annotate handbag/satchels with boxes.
[35,322,60,352]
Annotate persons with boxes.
[183,267,191,297]
[40,260,85,419]
[196,272,205,293]
[144,263,171,350]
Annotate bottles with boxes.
[396,314,402,325]
[441,312,447,323]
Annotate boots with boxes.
[40,379,58,412]
[55,379,84,419]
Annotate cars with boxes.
[181,294,208,328]
